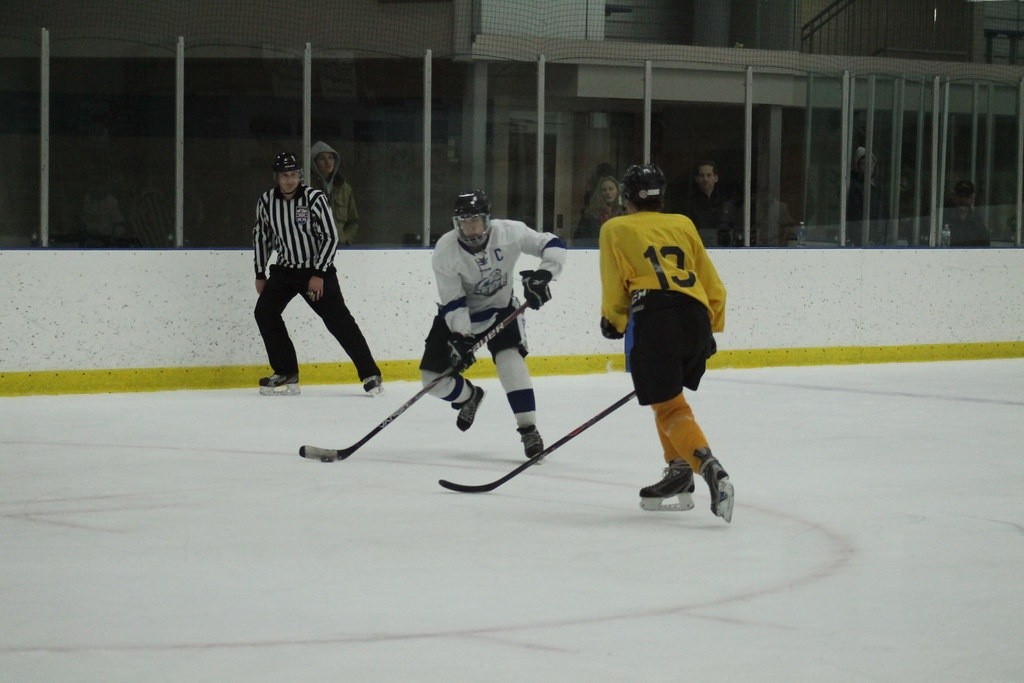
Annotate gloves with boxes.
[706,335,717,359]
[447,333,478,369]
[601,316,625,340]
[519,270,553,310]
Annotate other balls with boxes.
[321,456,334,462]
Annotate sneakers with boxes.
[639,459,696,510]
[362,375,385,397]
[694,447,735,522]
[516,425,546,465]
[258,373,301,395]
[451,379,486,432]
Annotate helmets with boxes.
[273,153,303,172]
[452,190,492,249]
[624,163,667,203]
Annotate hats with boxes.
[953,181,974,195]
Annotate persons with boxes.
[718,171,794,248]
[81,173,126,249]
[573,162,617,238]
[418,189,567,463]
[599,163,735,524]
[253,151,385,398]
[838,146,893,222]
[683,161,730,229]
[920,180,990,248]
[312,141,359,248]
[579,176,625,237]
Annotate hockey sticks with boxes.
[437,390,638,494]
[298,299,530,461]
